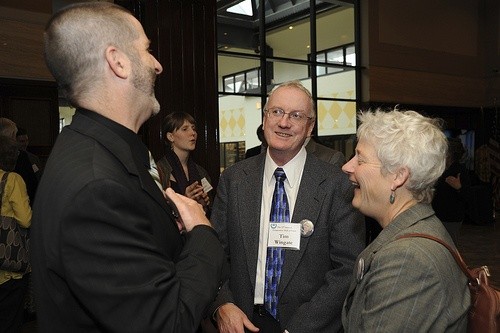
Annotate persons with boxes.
[31,2,228,333]
[156,110,213,217]
[0,118,45,333]
[431,136,468,257]
[341,108,472,333]
[245,124,346,168]
[208,81,366,333]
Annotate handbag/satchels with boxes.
[0,170,32,275]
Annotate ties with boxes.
[262,167,291,324]
[148,148,175,204]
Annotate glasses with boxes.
[263,106,313,127]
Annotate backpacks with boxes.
[382,231,500,333]
